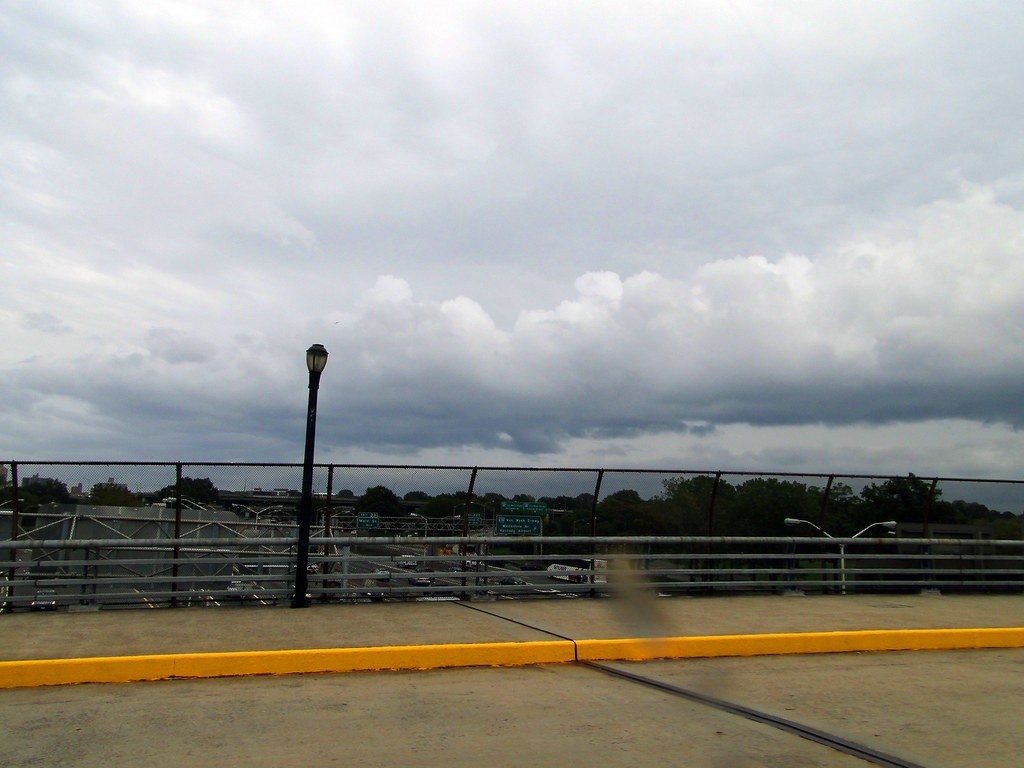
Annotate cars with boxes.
[407,576,431,585]
[31,590,59,610]
[370,568,391,582]
[498,577,526,585]
[350,529,359,534]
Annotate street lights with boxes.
[289,341,330,609]
[782,518,899,595]
[410,512,429,566]
[231,504,283,531]
[470,501,496,536]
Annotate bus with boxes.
[546,557,607,585]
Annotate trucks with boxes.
[397,555,417,568]
